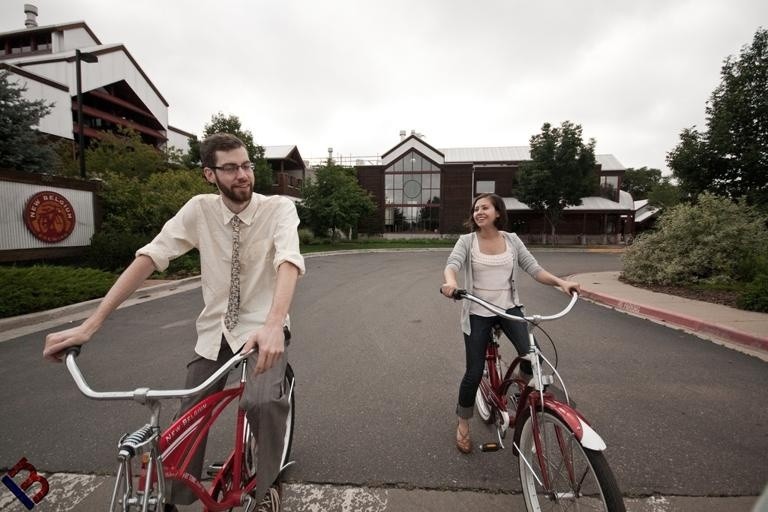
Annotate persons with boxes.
[441,193,582,453]
[43,132,306,512]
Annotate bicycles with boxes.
[437,286,627,512]
[53,321,299,511]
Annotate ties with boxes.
[224,214,240,334]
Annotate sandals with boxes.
[456,421,472,455]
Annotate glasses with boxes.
[209,160,257,174]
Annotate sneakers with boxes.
[252,481,285,512]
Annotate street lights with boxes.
[73,49,98,177]
